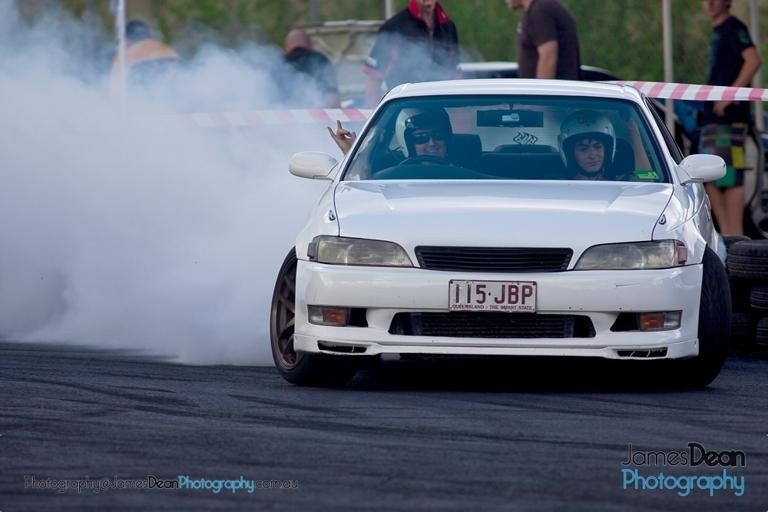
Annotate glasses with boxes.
[410,130,449,144]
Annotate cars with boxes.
[270,78,731,390]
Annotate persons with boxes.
[326,115,453,169]
[363,0,460,110]
[694,1,764,237]
[109,20,187,96]
[268,27,339,109]
[557,110,632,181]
[505,0,582,81]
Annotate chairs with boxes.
[448,133,638,181]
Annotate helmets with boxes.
[556,107,618,173]
[394,104,453,158]
